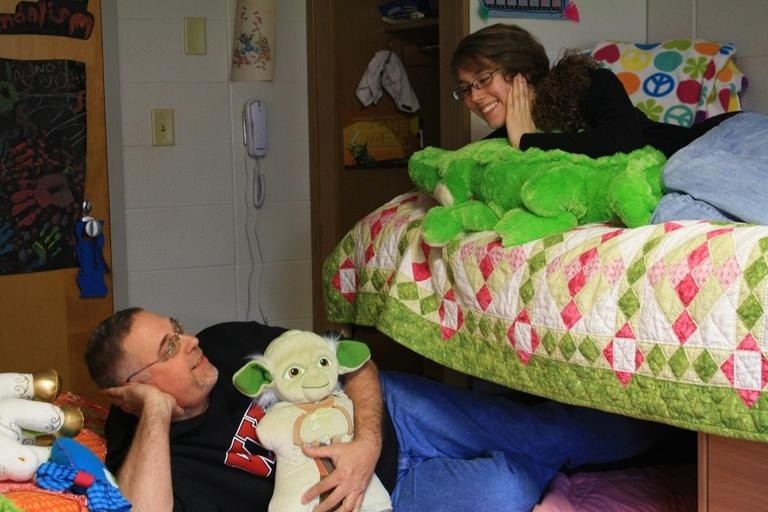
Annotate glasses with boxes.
[126,318,185,383]
[448,66,503,102]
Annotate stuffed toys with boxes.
[0,363,83,482]
[401,137,665,251]
[230,329,393,512]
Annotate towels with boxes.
[354,49,421,114]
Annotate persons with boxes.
[446,21,766,225]
[83,305,669,512]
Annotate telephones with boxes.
[245,98,267,158]
